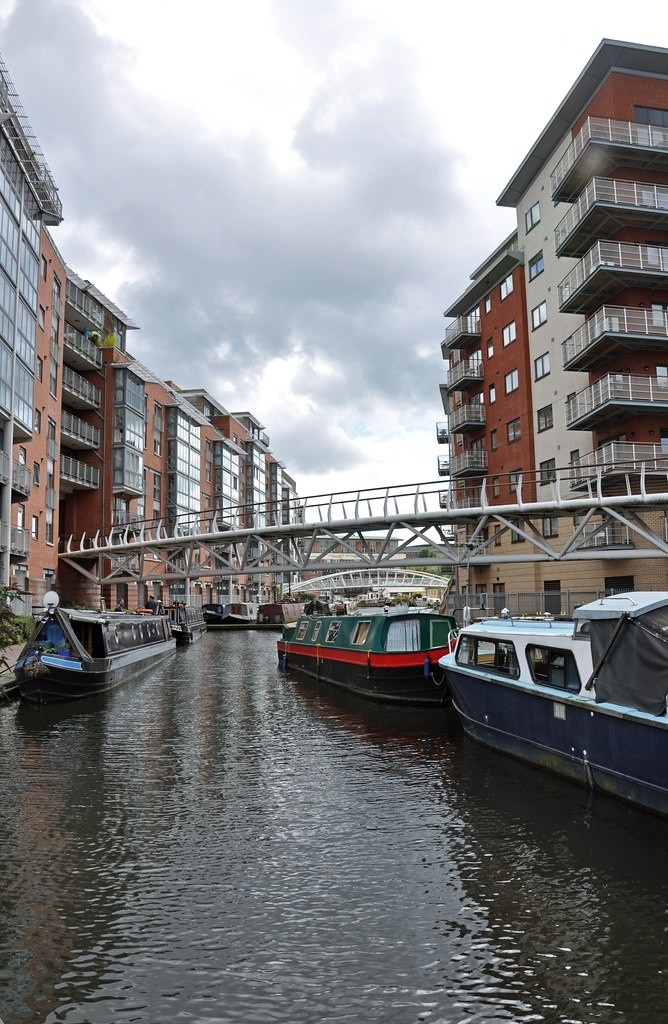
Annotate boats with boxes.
[276,609,460,708]
[160,605,207,646]
[440,587,668,819]
[302,588,441,616]
[12,603,177,706]
[201,602,283,623]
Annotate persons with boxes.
[145,594,158,615]
[384,604,389,614]
[114,597,125,612]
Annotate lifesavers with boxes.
[135,608,154,613]
